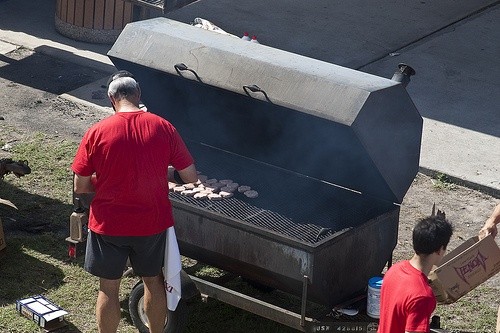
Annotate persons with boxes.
[71,69,200,332]
[479,202,500,242]
[376,214,453,332]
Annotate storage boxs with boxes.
[16,293,69,333]
[426,231,500,304]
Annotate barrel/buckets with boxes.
[366,276,384,319]
[69,207,88,242]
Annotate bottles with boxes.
[242,32,251,41]
[250,35,259,43]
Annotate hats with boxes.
[105,69,142,111]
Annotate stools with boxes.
[65,237,87,259]
